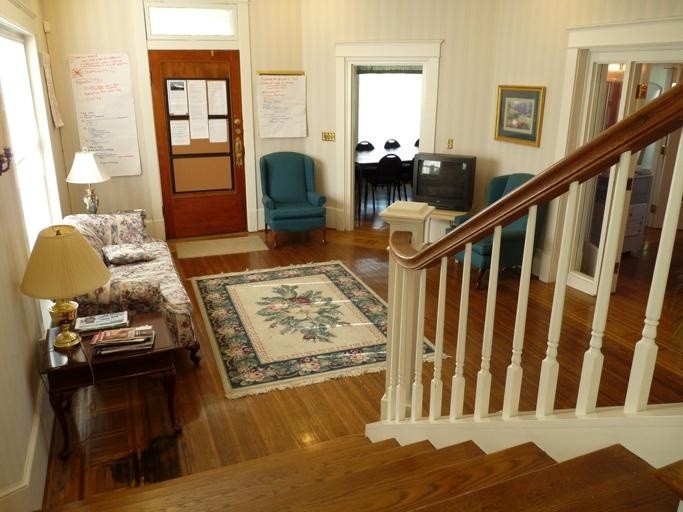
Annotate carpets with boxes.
[190,259,452,399]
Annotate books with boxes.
[89,326,155,355]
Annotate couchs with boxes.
[62,213,200,371]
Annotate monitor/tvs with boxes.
[411,153,476,212]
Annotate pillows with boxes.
[102,244,153,263]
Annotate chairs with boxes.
[355,141,374,150]
[384,139,400,148]
[415,139,419,146]
[393,156,414,203]
[260,152,327,248]
[364,154,403,216]
[453,172,548,290]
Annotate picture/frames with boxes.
[494,84,547,148]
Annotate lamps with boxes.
[21,225,113,352]
[65,151,109,215]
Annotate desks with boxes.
[38,313,182,459]
[424,209,468,244]
[589,172,655,257]
[354,146,419,226]
